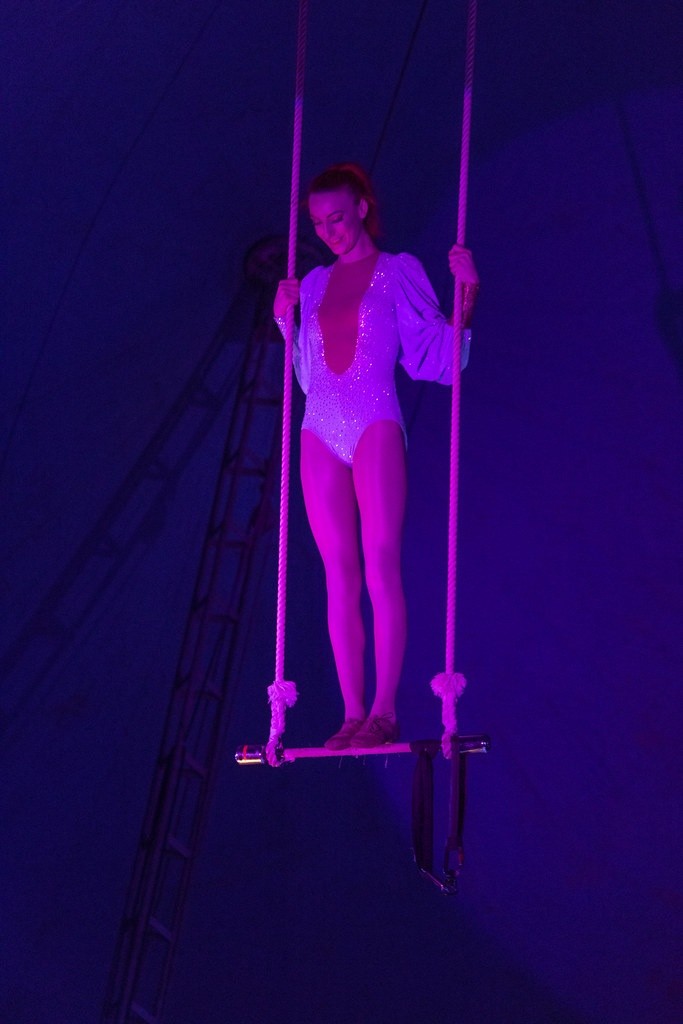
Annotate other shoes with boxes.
[349,712,398,747]
[323,718,365,750]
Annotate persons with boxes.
[273,164,480,746]
